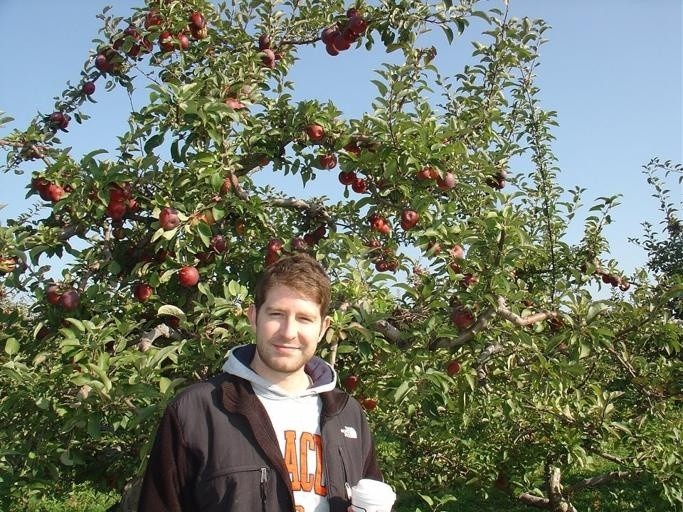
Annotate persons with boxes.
[136,254,385,512]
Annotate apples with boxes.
[0,0,508,411]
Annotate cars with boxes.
[351,478,397,512]
